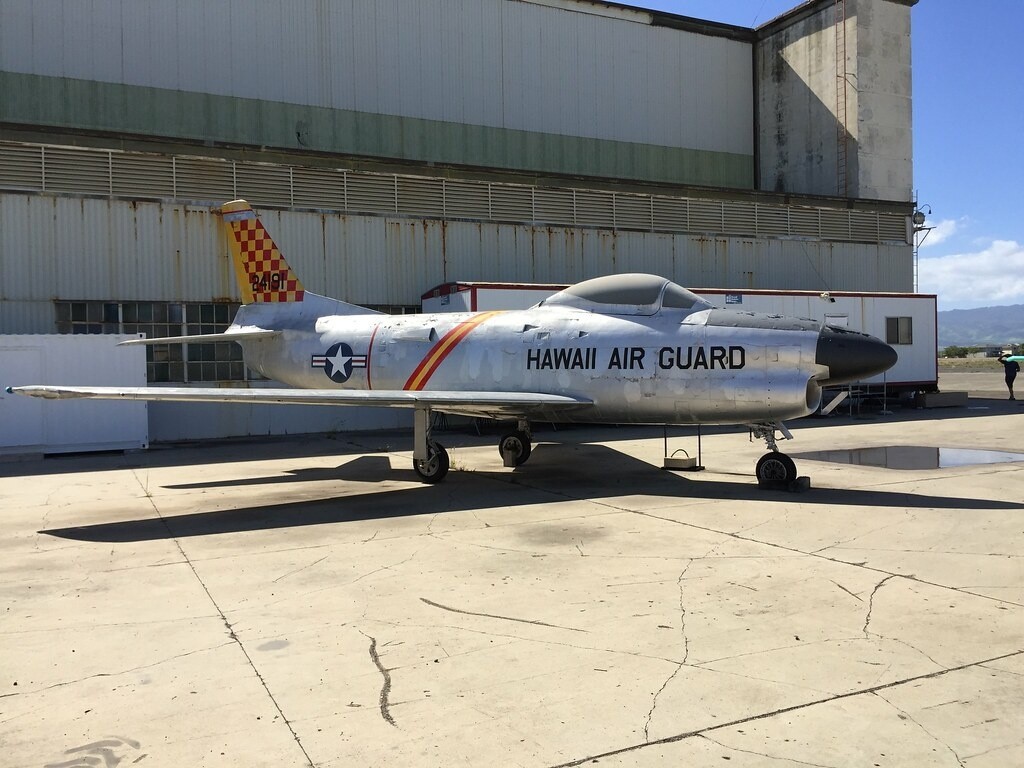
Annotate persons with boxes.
[998,354,1020,400]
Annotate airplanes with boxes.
[6,198,898,491]
